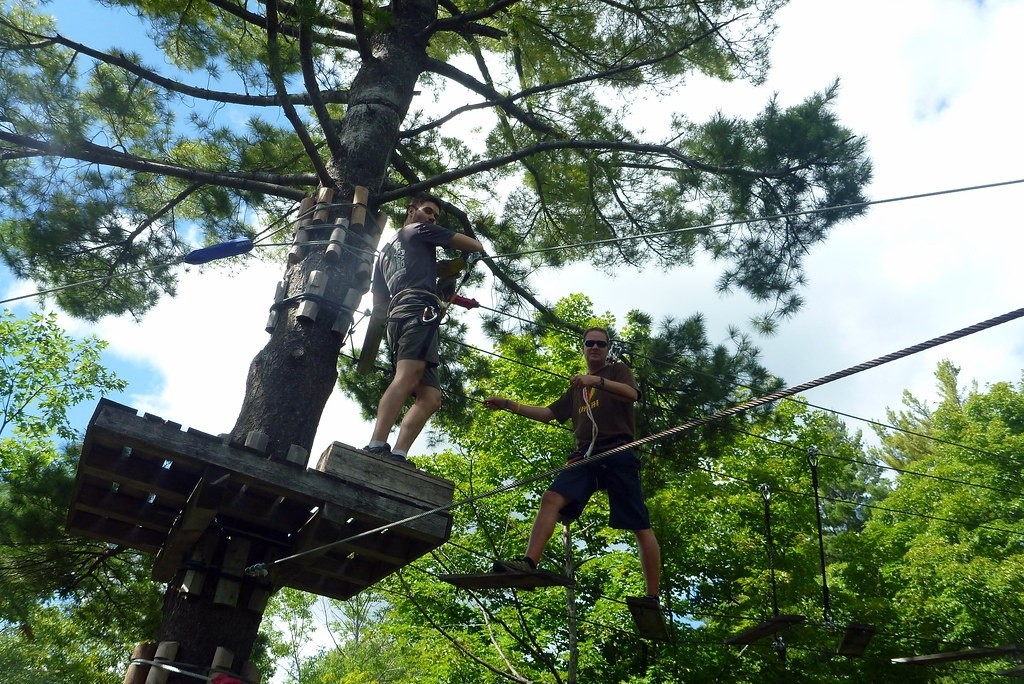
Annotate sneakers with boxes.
[494,557,536,576]
[363,444,417,470]
[642,595,662,612]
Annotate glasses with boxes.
[584,340,609,348]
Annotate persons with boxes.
[484,327,668,623]
[361,193,486,469]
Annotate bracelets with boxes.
[597,377,605,388]
[505,399,509,407]
[515,403,520,413]
